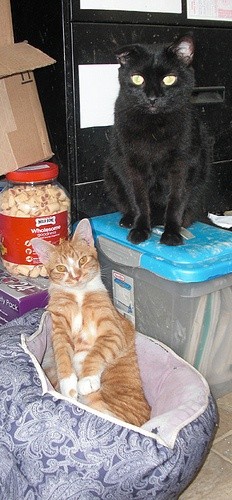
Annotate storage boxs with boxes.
[0,259,49,326]
[0,2,58,180]
[71,206,231,399]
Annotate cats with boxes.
[102,32,214,246]
[30,218,152,427]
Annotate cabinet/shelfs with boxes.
[60,0,232,221]
